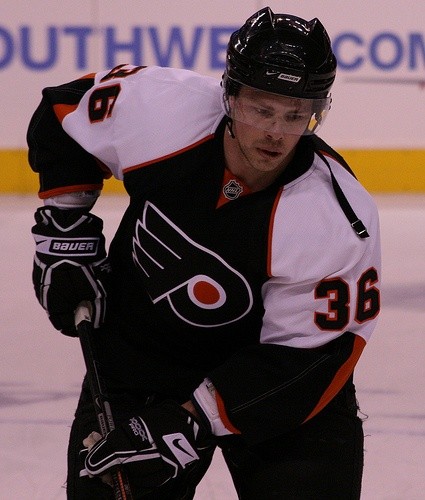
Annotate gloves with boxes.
[79,407,215,500]
[31,206,106,336]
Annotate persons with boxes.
[26,6,382,500]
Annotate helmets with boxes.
[220,7,337,134]
